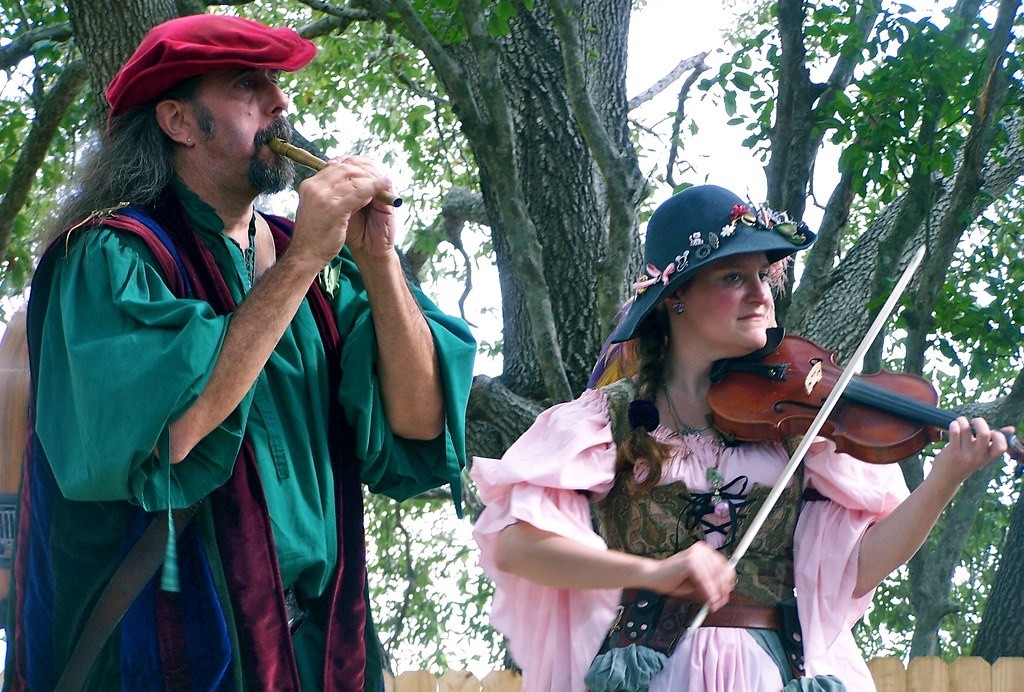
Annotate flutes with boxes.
[269,136,404,208]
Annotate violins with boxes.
[708,326,1023,465]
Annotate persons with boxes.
[468,184,1014,691]
[0,13,475,692]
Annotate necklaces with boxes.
[227,202,256,290]
[663,382,725,447]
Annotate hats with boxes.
[105,14,317,138]
[610,184,819,345]
